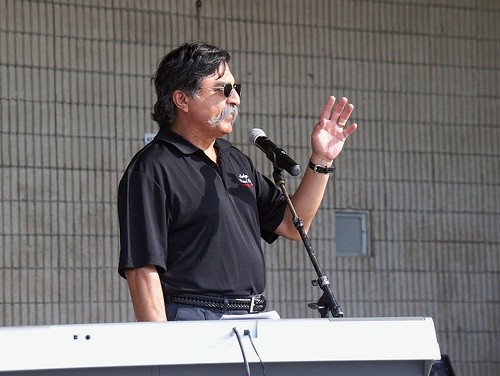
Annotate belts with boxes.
[165,291,267,313]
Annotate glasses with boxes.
[197,83,242,98]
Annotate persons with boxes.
[115,41,358,321]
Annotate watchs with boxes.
[309,159,337,175]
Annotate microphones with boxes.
[248,128,301,176]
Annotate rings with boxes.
[337,120,345,126]
